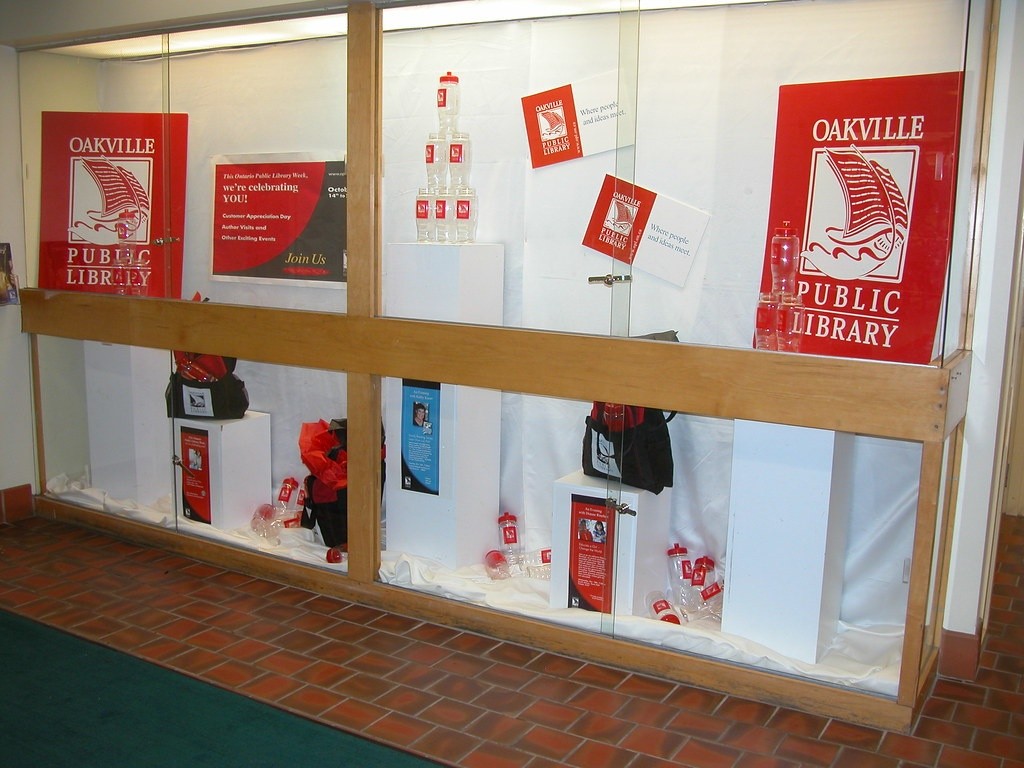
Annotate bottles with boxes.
[771,221,799,297]
[416,187,436,243]
[117,210,136,261]
[437,71,461,134]
[692,556,715,607]
[111,260,129,296]
[435,186,457,244]
[456,187,478,244]
[668,543,695,613]
[252,476,308,537]
[325,543,348,563]
[694,577,725,618]
[646,591,681,626]
[425,132,448,189]
[755,292,779,351]
[449,132,472,188]
[777,294,804,352]
[485,512,552,580]
[130,260,146,297]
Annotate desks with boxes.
[550,469,670,620]
[172,409,272,529]
